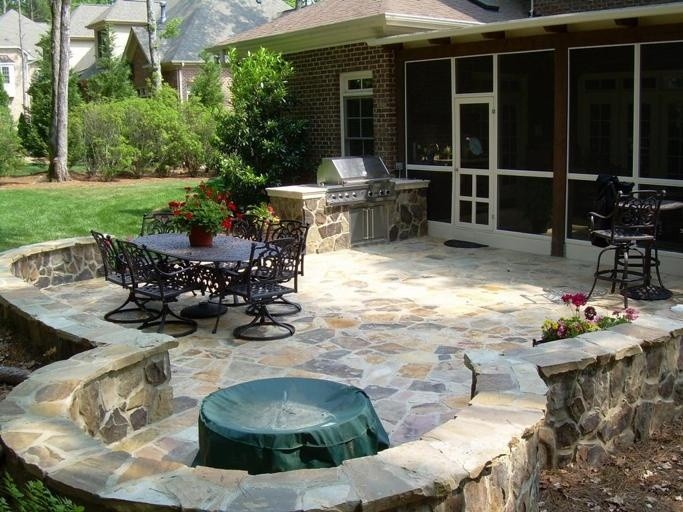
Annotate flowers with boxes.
[161,184,234,234]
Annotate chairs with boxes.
[139,214,181,302]
[116,240,197,337]
[587,190,672,309]
[208,207,256,306]
[244,218,310,316]
[221,238,302,340]
[90,230,161,322]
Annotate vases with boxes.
[190,224,213,247]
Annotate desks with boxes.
[613,200,683,300]
[132,234,282,319]
